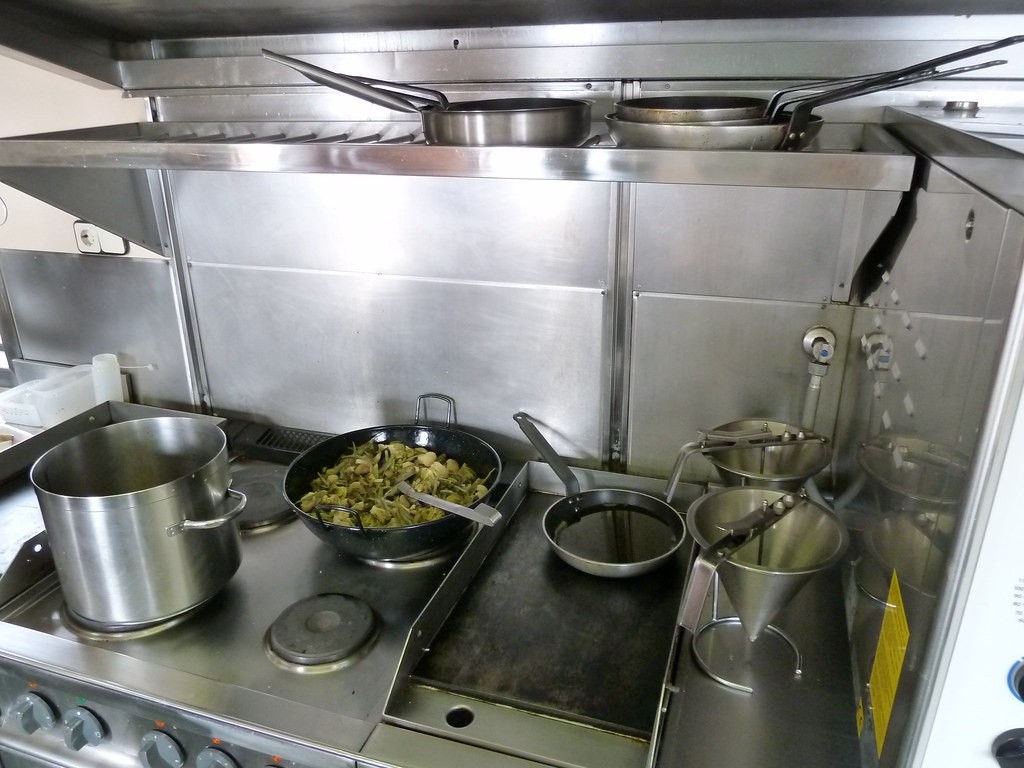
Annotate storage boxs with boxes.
[0,378,42,427]
[29,363,97,430]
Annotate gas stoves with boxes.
[0,455,503,768]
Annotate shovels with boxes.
[382,471,502,527]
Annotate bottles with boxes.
[93,354,123,405]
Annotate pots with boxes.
[605,35,1024,150]
[284,394,502,559]
[513,412,686,576]
[257,49,591,148]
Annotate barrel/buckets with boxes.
[31,416,247,625]
